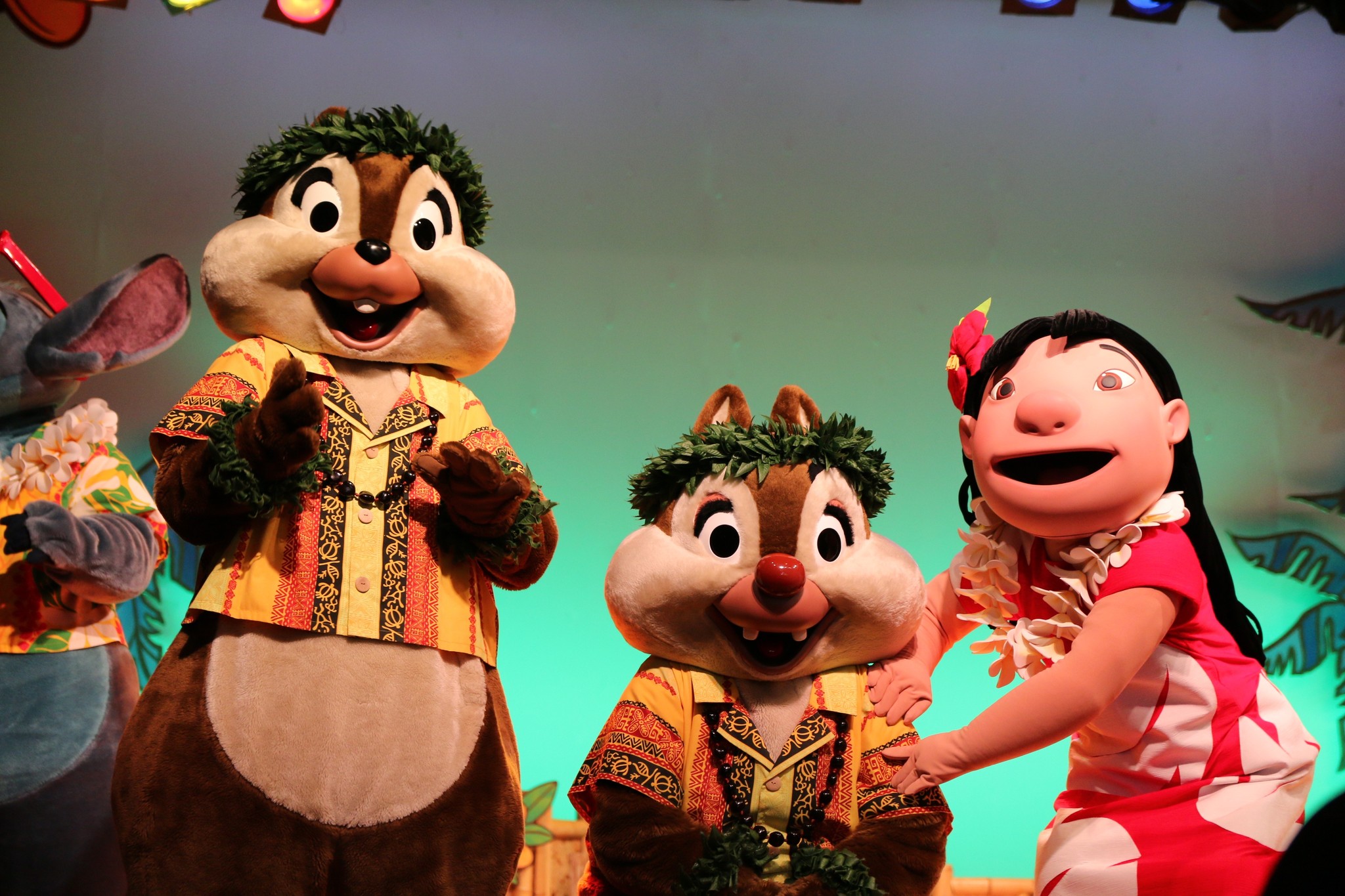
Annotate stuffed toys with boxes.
[568,385,954,896]
[0,105,559,896]
[866,299,1321,896]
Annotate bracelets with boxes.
[205,394,330,518]
[683,824,891,896]
[437,452,557,571]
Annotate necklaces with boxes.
[955,490,1186,688]
[1,398,119,500]
[702,702,849,847]
[315,407,438,505]
